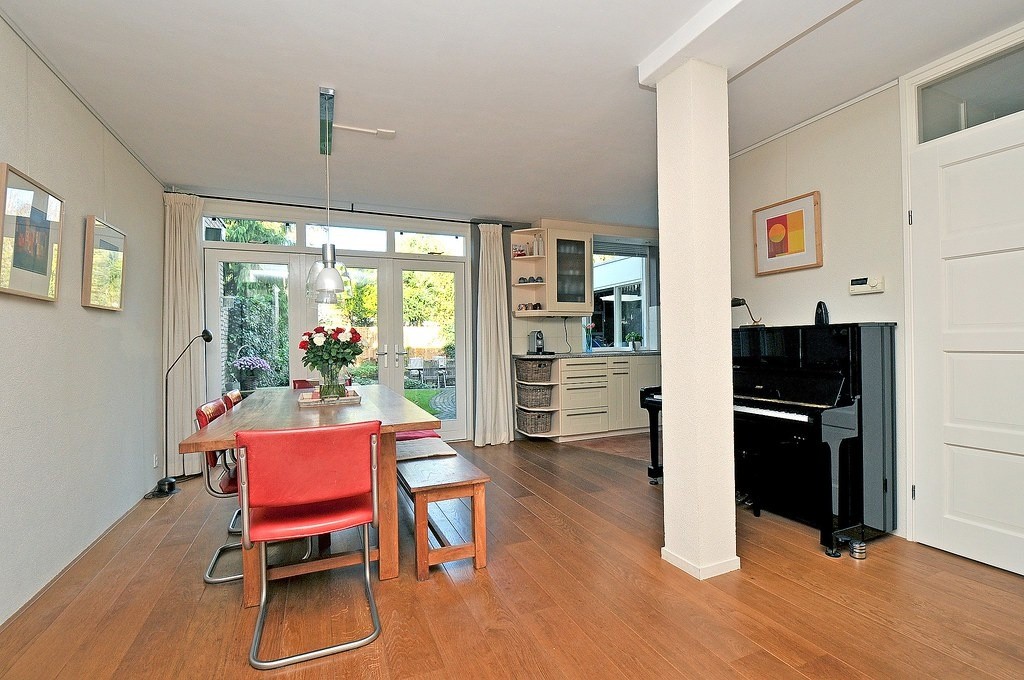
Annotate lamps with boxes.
[303,97,355,304]
[154,329,213,497]
[731,297,763,326]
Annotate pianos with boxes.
[640,319,898,558]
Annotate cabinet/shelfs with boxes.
[559,358,608,436]
[510,227,549,313]
[513,357,559,439]
[628,356,660,428]
[607,357,629,430]
[549,231,595,315]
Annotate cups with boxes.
[517,277,544,311]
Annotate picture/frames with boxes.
[750,191,821,275]
[0,162,65,302]
[81,215,127,311]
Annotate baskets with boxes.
[515,359,551,383]
[516,383,552,407]
[517,408,552,434]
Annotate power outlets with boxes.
[154,453,159,468]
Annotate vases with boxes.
[320,365,348,399]
[630,340,641,350]
[587,333,592,353]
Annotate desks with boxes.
[178,384,440,608]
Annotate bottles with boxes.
[524,238,544,255]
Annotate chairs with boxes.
[196,399,314,585]
[292,376,352,389]
[224,389,249,464]
[236,417,384,669]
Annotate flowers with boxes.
[581,323,596,336]
[627,332,644,342]
[298,325,365,375]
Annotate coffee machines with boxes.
[527,331,555,355]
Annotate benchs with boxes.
[395,431,491,583]
